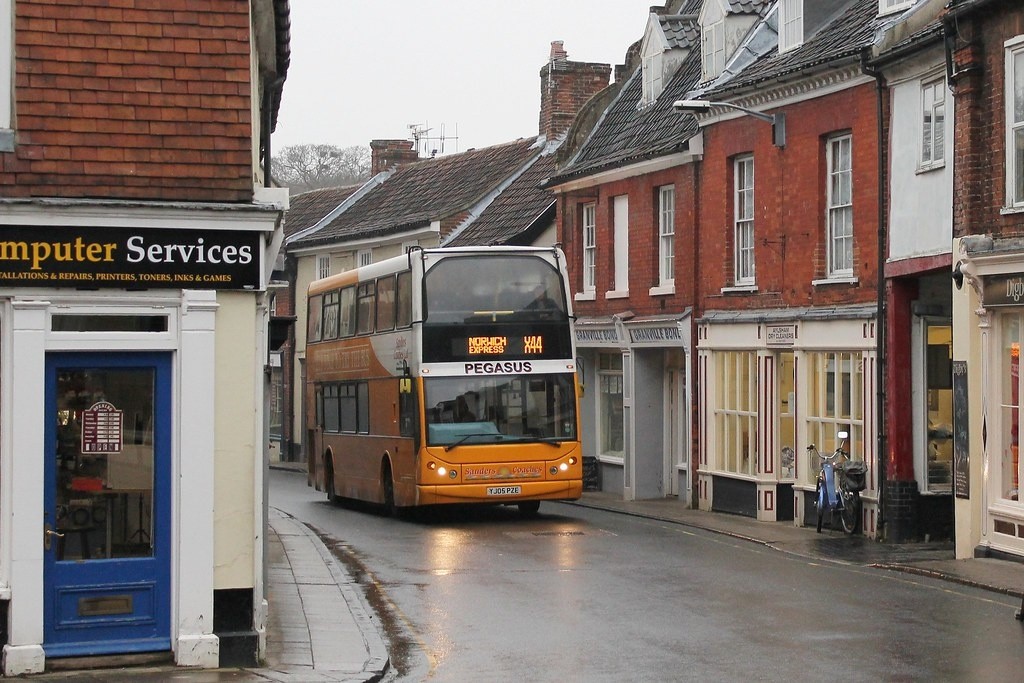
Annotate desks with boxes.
[67,484,151,559]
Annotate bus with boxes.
[304,242,586,509]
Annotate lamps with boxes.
[674,100,786,145]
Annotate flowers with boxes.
[781,445,795,468]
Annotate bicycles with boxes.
[807,430,863,536]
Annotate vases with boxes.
[782,468,790,477]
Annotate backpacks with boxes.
[839,460,868,492]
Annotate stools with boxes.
[57,525,96,561]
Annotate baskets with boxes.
[809,452,839,471]
